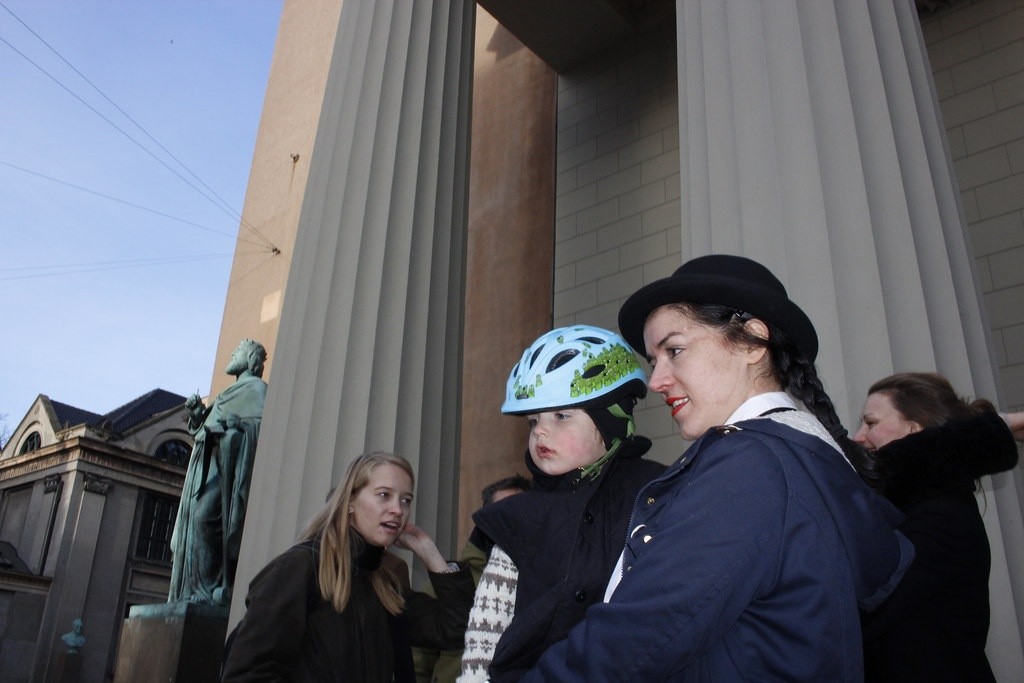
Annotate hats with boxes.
[617,254,818,364]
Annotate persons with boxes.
[411,471,531,683]
[517,254,916,683]
[852,372,1019,683]
[167,336,268,604]
[455,324,670,683]
[220,451,476,683]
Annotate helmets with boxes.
[501,325,649,416]
